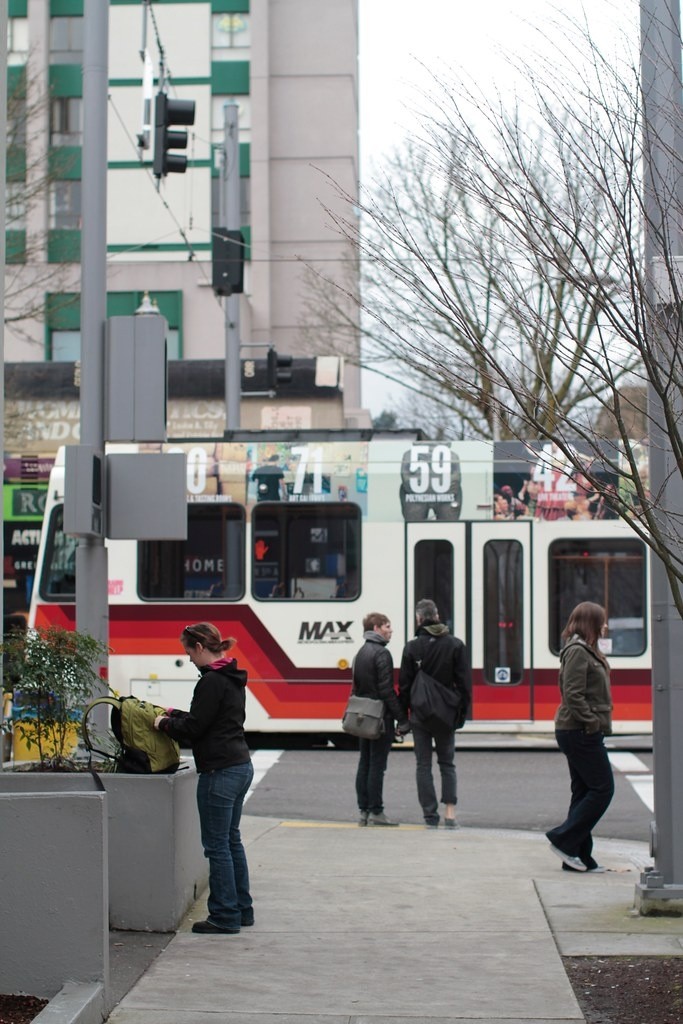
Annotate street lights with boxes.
[491,272,619,441]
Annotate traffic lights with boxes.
[136,85,195,179]
[267,348,295,390]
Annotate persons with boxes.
[495,440,629,521]
[543,602,615,872]
[354,613,409,826]
[398,599,474,830]
[153,621,255,934]
[250,455,286,502]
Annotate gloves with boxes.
[398,719,412,736]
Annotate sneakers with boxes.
[549,841,587,872]
[588,865,607,873]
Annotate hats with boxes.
[268,454,279,462]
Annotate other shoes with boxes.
[357,811,400,827]
[425,820,439,829]
[444,818,460,830]
[192,921,240,934]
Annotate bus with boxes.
[20,441,682,751]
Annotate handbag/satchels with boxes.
[341,693,387,740]
[410,669,462,736]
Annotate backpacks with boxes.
[81,694,190,791]
